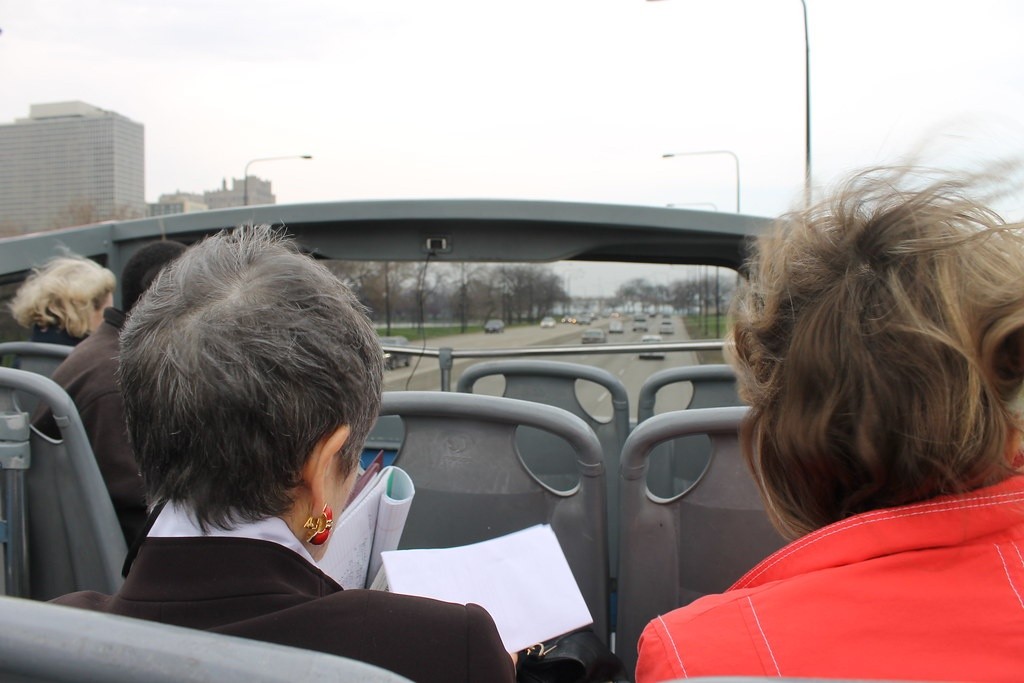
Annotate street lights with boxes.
[663,149,741,214]
[646,0,813,220]
[243,155,314,206]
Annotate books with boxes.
[312,447,415,591]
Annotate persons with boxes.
[44,224,517,683]
[634,166,1024,683]
[8,241,188,551]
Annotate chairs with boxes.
[0,341,809,683]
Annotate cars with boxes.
[639,335,666,360]
[581,327,606,343]
[484,318,506,334]
[659,319,675,335]
[631,316,650,331]
[607,319,625,333]
[377,335,413,371]
[540,316,556,328]
[559,308,674,326]
